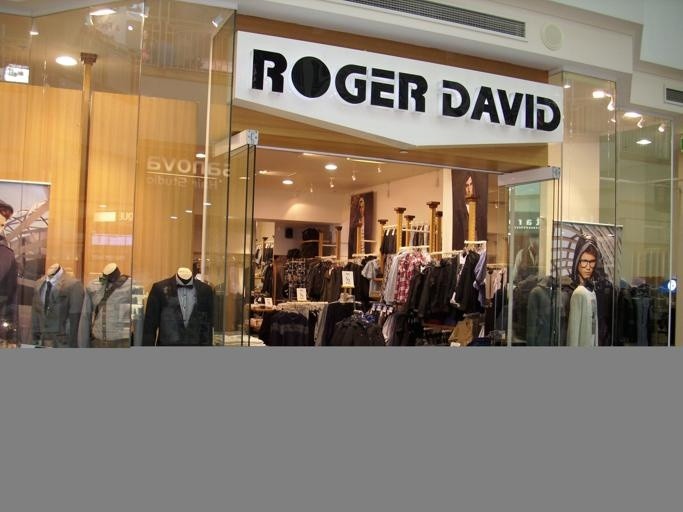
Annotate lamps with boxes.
[55,55,77,68]
[635,138,652,146]
[658,122,667,134]
[211,14,223,28]
[29,29,40,38]
[281,179,293,186]
[622,110,644,128]
[125,24,133,31]
[591,90,614,112]
[195,151,205,160]
[562,80,574,88]
[88,6,117,16]
[324,163,337,173]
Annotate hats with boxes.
[0,199,13,213]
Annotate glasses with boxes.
[579,259,598,269]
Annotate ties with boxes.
[43,281,52,316]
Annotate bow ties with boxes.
[177,283,193,290]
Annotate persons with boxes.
[349,197,372,255]
[0,197,18,343]
[73,262,144,348]
[559,236,613,346]
[29,261,84,348]
[456,173,485,248]
[142,265,216,345]
[514,232,540,285]
